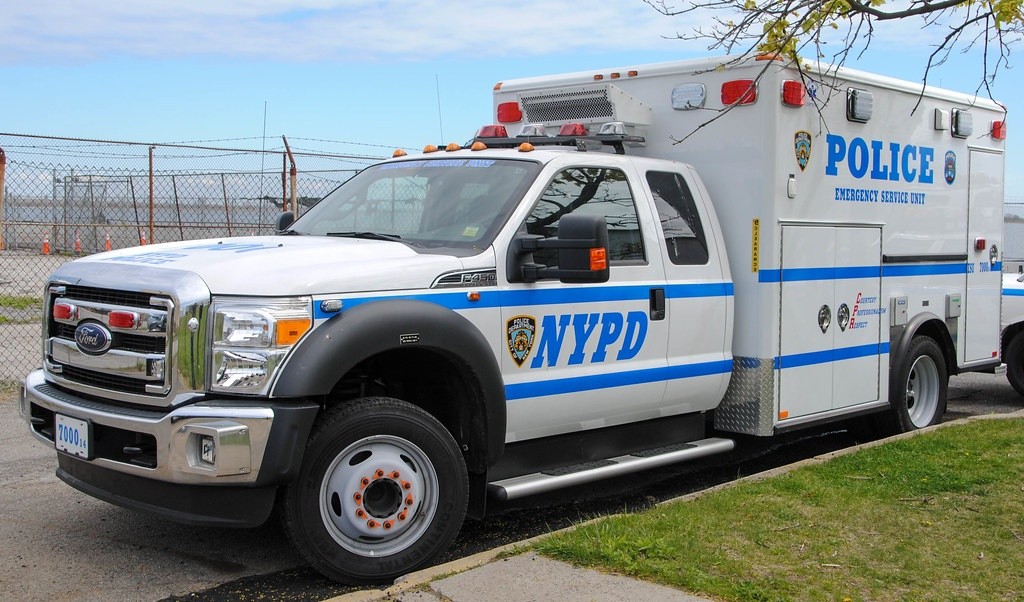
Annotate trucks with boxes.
[1003,272,1023,401]
[15,48,1006,586]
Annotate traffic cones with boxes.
[74,232,82,252]
[104,230,111,252]
[140,226,147,245]
[39,232,54,255]
